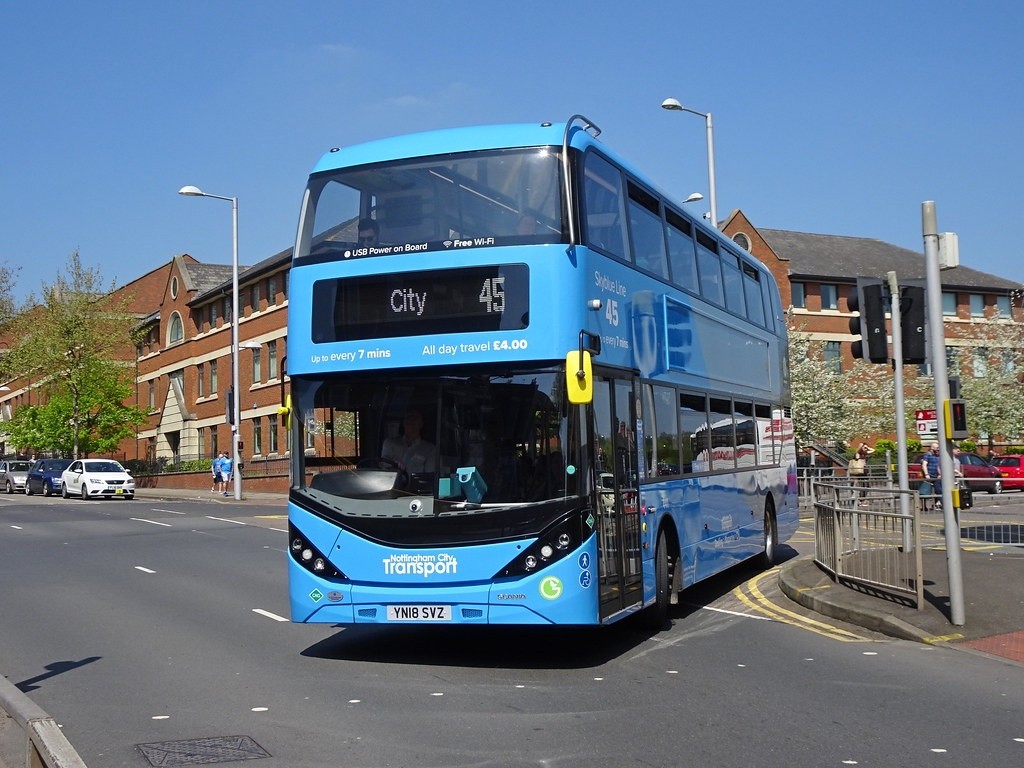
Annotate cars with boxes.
[991,455,1024,492]
[25,459,75,495]
[596,472,617,514]
[908,451,1003,494]
[59,459,136,500]
[0,460,35,494]
[650,462,680,475]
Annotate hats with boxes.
[224,451,229,454]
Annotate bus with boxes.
[688,415,761,473]
[278,115,796,629]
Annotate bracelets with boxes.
[925,474,929,476]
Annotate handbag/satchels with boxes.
[918,481,934,494]
[848,459,865,475]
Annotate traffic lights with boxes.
[846,285,888,366]
[945,400,968,441]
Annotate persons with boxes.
[988,448,995,458]
[358,218,380,249]
[920,442,963,511]
[381,408,436,489]
[516,215,537,235]
[853,442,875,507]
[211,451,234,497]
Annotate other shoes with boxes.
[218,491,222,495]
[223,491,228,497]
[211,487,214,493]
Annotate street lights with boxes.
[924,231,969,625]
[659,98,719,229]
[179,186,243,500]
